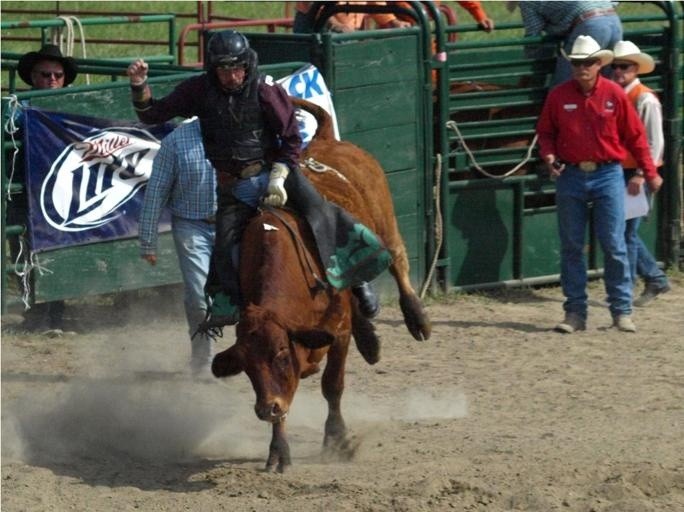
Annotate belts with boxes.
[559,158,618,170]
[570,7,616,29]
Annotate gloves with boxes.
[261,162,290,209]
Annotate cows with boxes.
[432,73,553,209]
[211,95,432,474]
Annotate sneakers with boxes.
[21,313,79,339]
[601,277,674,307]
[553,316,588,334]
[612,314,637,333]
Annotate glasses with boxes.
[32,69,64,79]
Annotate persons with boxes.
[289,0,411,35]
[498,1,626,174]
[375,0,494,35]
[135,116,223,385]
[10,42,84,339]
[603,36,672,310]
[531,33,663,337]
[125,29,379,320]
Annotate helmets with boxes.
[202,29,259,96]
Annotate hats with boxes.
[559,35,614,75]
[612,40,656,75]
[17,44,79,86]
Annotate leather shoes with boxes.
[351,282,380,318]
